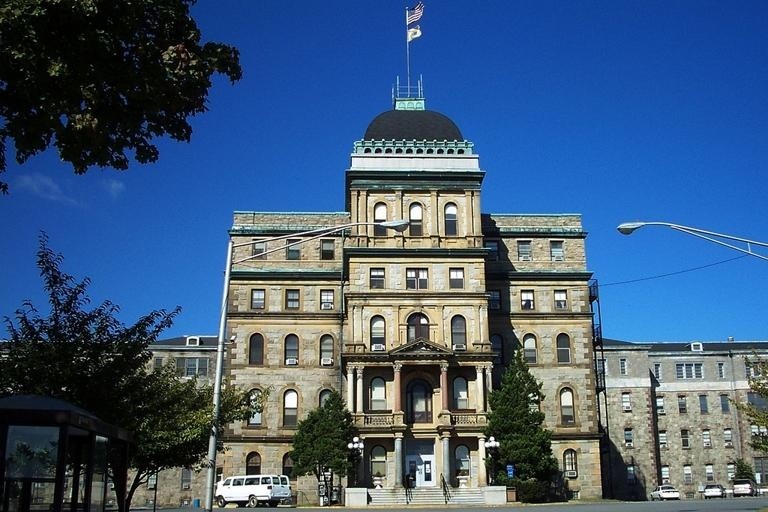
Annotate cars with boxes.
[732,478,757,497]
[702,484,727,499]
[649,485,681,501]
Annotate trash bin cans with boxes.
[194,499,200,508]
[507,487,516,502]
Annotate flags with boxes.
[408,24,423,43]
[407,2,426,26]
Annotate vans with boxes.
[215,474,292,508]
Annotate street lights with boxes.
[347,436,365,486]
[484,436,500,486]
[203,219,411,510]
[615,219,767,261]
